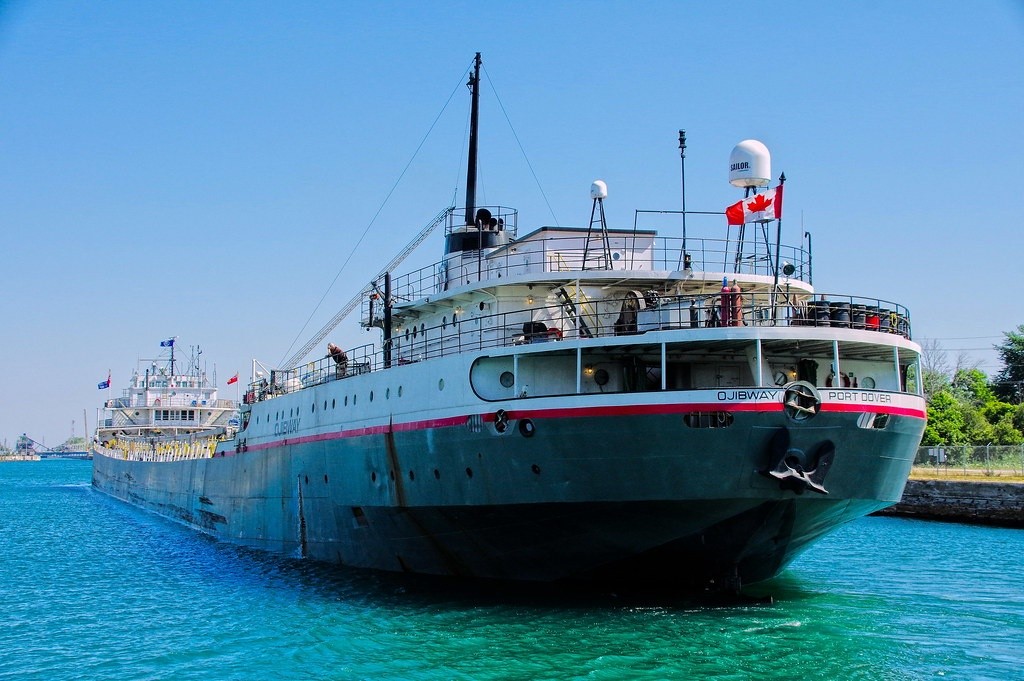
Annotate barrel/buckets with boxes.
[808,301,910,341]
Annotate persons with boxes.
[328,342,348,376]
[261,377,268,400]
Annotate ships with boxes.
[86,47,929,609]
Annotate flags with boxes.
[227,374,239,385]
[160,339,175,347]
[98,379,110,389]
[725,184,784,226]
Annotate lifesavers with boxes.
[825,371,851,388]
